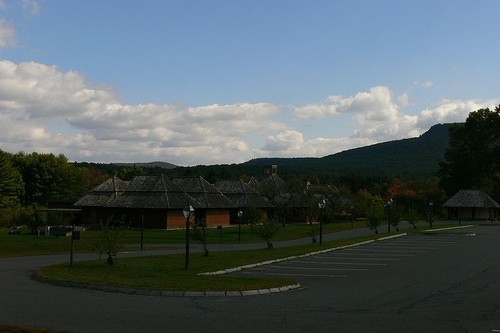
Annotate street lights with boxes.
[386,197,393,234]
[429,199,434,229]
[317,196,327,247]
[458,201,462,225]
[350,202,355,230]
[182,200,194,269]
[236,209,243,243]
